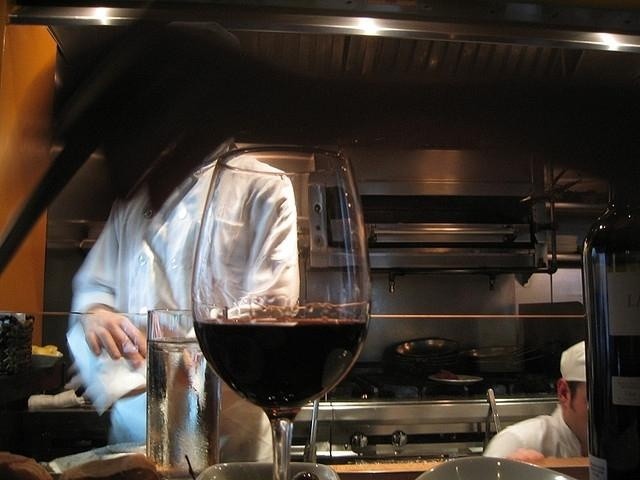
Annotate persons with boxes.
[60,21,302,466]
[479,340,590,462]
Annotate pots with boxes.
[460,344,530,372]
[383,336,465,376]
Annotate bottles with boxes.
[577,149,639,480]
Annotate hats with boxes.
[560,340,587,383]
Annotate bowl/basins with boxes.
[410,456,581,480]
[31,352,65,367]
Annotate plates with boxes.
[428,373,484,383]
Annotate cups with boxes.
[144,307,222,474]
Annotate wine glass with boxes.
[190,146,373,478]
